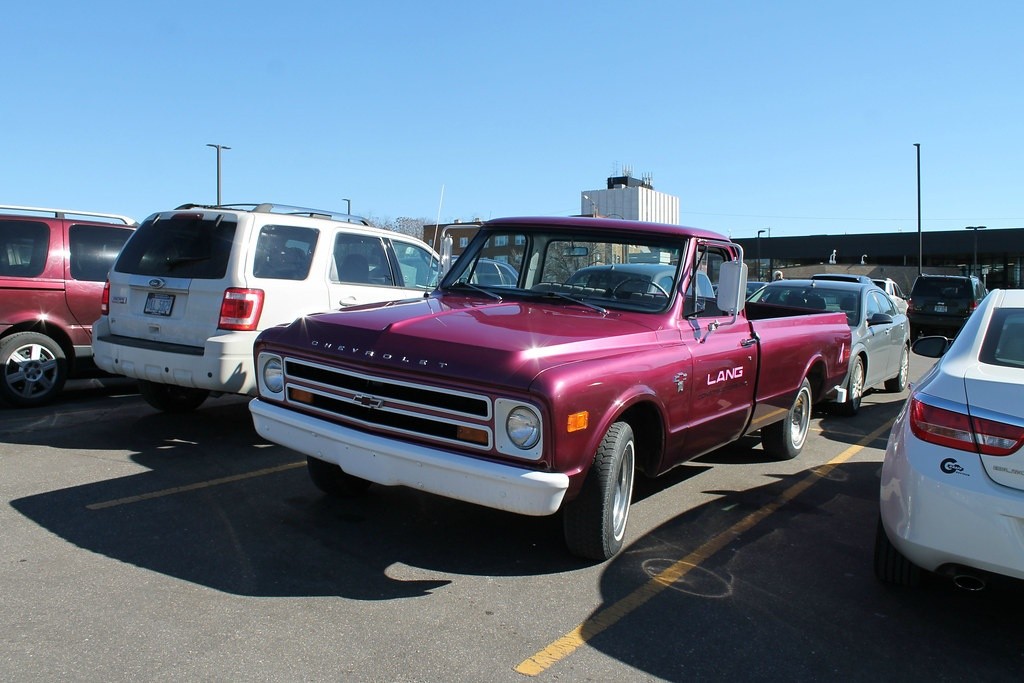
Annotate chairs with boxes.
[279,247,306,277]
[840,296,856,310]
[807,297,827,309]
[929,285,941,295]
[340,253,369,284]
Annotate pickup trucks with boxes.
[248,216,855,562]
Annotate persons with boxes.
[773,270,784,280]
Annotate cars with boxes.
[565,263,716,299]
[744,278,911,413]
[874,289,1024,592]
[809,274,878,305]
[433,256,518,289]
[712,283,783,301]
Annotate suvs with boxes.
[871,279,909,317]
[905,272,990,344]
[0,204,141,408]
[91,202,444,413]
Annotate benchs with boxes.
[531,281,669,307]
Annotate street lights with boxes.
[343,199,350,223]
[914,143,922,275]
[207,143,232,207]
[965,226,987,276]
[757,230,765,280]
